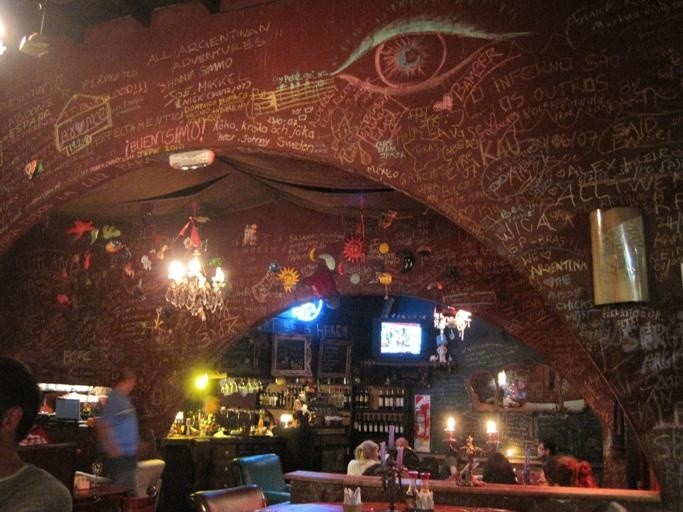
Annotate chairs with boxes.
[228,453,291,503]
[188,487,266,512]
[109,483,161,511]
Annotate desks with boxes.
[254,500,512,512]
[72,468,132,511]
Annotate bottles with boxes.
[354,387,369,409]
[258,382,300,408]
[353,412,405,434]
[377,387,404,410]
[179,406,266,440]
[403,469,431,510]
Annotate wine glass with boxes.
[219,376,263,397]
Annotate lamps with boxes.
[162,201,228,318]
[442,414,500,485]
[17,0,56,60]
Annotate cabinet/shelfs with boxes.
[256,371,412,472]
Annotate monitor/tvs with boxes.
[372,317,430,362]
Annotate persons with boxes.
[267,411,321,475]
[1,353,156,511]
[346,437,597,488]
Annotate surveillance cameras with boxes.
[418,247,432,257]
